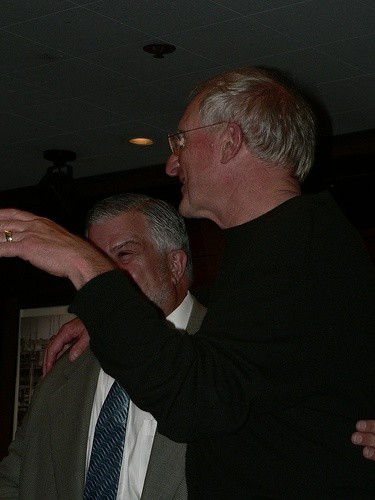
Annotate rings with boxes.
[4,231,12,243]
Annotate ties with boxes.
[82,379,131,500]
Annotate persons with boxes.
[0,66,375,500]
[0,194,375,500]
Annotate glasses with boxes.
[167,120,229,155]
